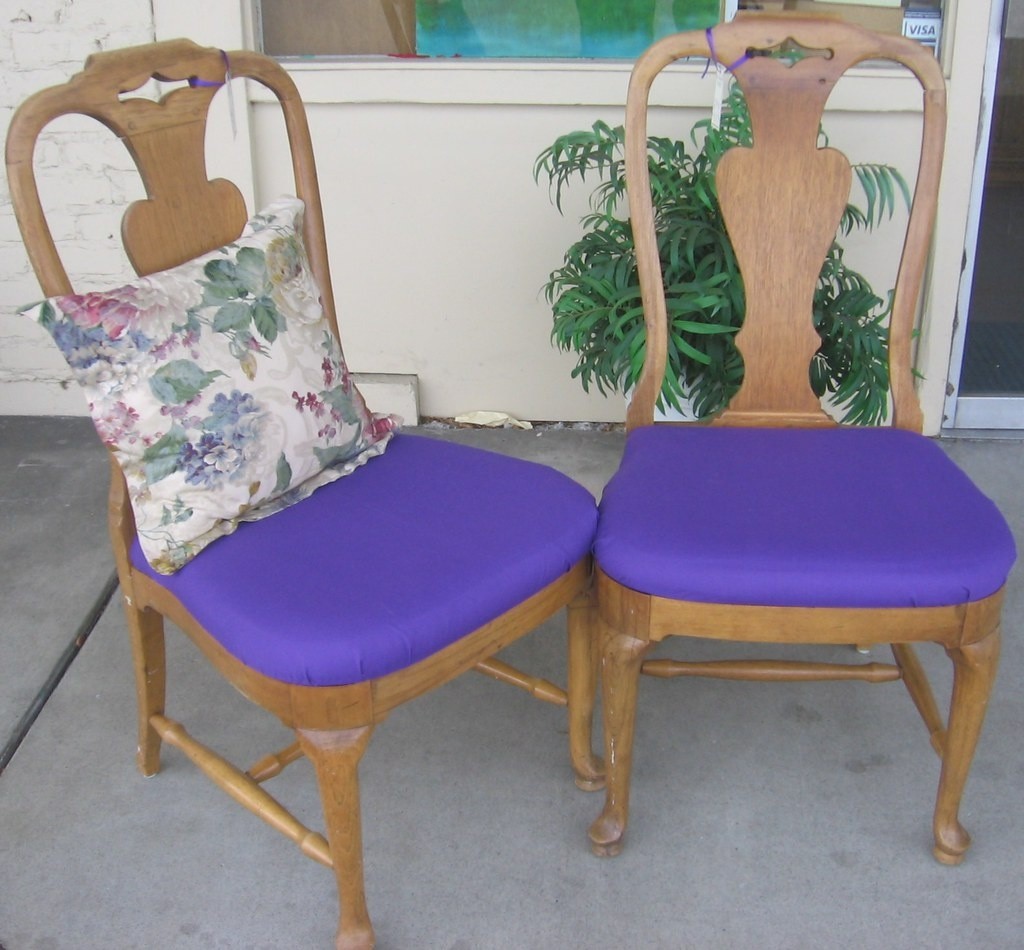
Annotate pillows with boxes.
[19,196,394,575]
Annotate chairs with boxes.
[585,20,1017,866]
[4,39,607,950]
